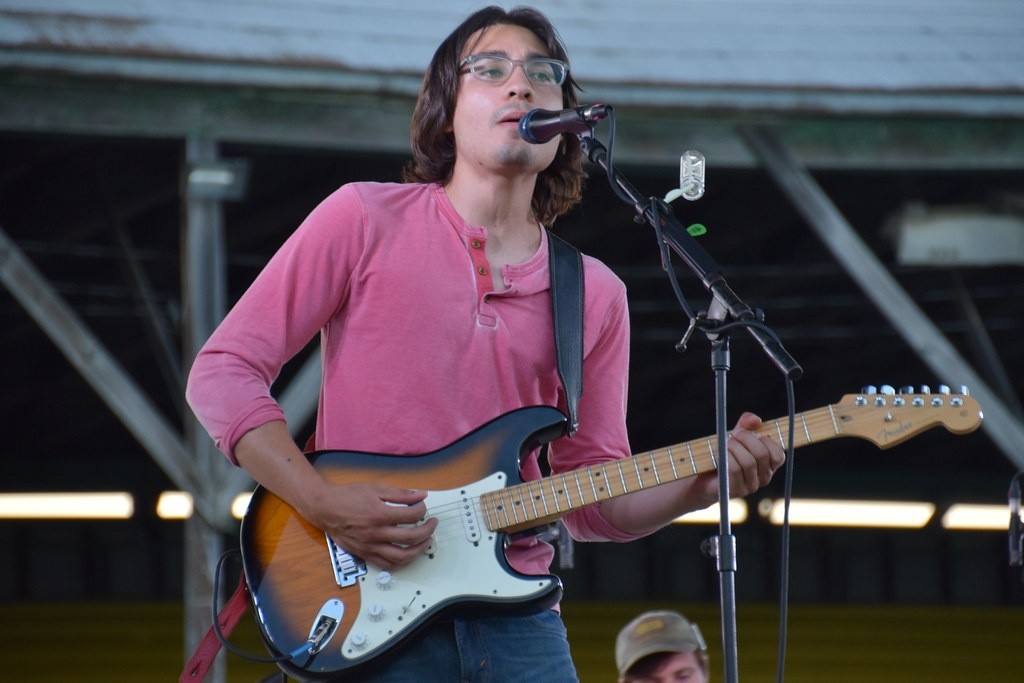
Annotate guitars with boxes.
[238,379,988,683]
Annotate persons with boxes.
[616,611,710,683]
[184,7,786,683]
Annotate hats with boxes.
[614,609,702,679]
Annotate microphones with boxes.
[518,102,613,144]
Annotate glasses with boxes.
[458,54,571,87]
